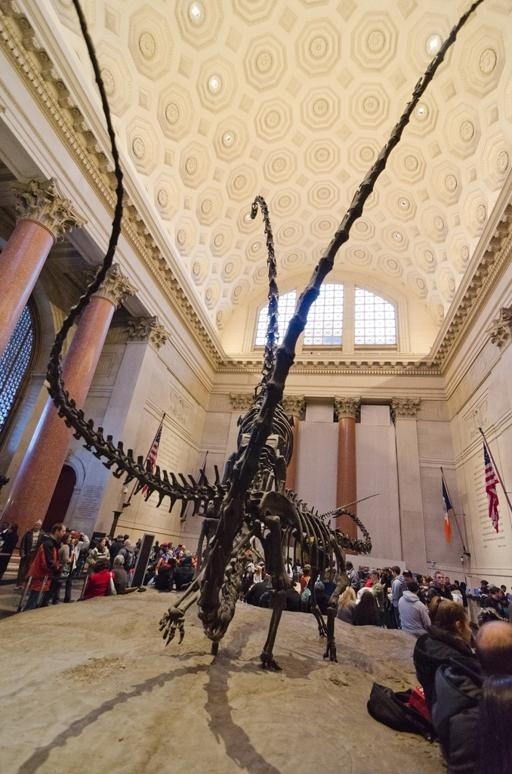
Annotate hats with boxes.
[403,569,411,575]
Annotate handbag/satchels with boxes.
[110,577,117,595]
[58,562,71,579]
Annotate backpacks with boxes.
[125,549,135,569]
[367,682,434,742]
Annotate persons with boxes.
[470,672,511,774]
[412,599,473,712]
[430,653,486,760]
[445,617,511,774]
[1,520,511,647]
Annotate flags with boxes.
[439,476,453,545]
[196,457,206,488]
[483,442,500,533]
[141,424,167,494]
